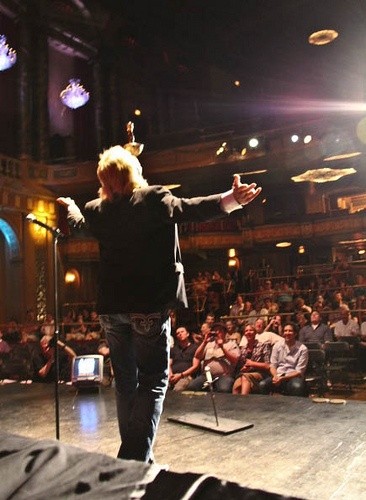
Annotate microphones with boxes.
[25,214,60,234]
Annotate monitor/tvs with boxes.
[72,355,103,383]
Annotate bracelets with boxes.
[181,372,184,378]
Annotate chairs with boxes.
[167,336,363,400]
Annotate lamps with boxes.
[322,136,362,162]
[309,30,338,45]
[123,120,144,157]
[216,137,246,157]
[60,78,90,109]
[65,272,75,283]
[0,35,16,71]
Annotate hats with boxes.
[211,322,227,331]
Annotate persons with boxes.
[57,143,262,466]
[0,304,117,389]
[256,321,309,395]
[186,323,240,391]
[168,246,366,373]
[168,326,200,391]
[232,317,272,396]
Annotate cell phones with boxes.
[210,332,218,337]
